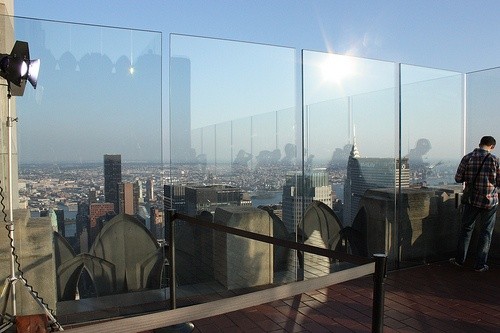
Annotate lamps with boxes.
[0,40,41,96]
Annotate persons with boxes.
[404,138,434,186]
[232,142,352,203]
[447,136,500,272]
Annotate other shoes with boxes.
[448,257,464,268]
[479,264,490,272]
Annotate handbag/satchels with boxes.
[461,183,478,207]
